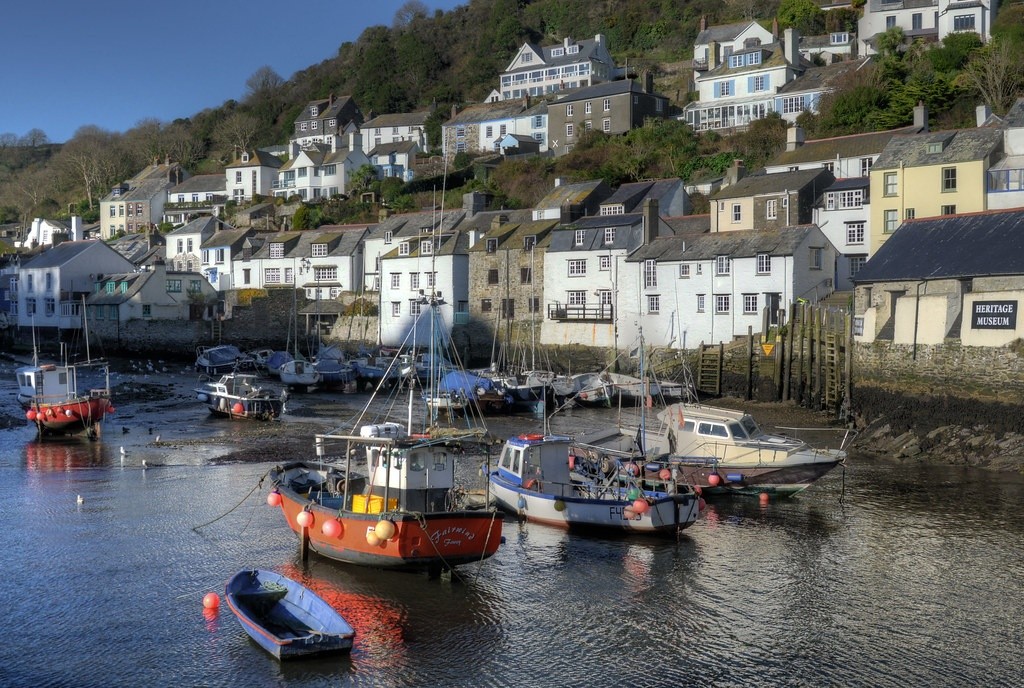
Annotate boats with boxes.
[479,244,706,540]
[26,397,115,436]
[568,350,860,517]
[16,311,73,405]
[194,246,692,419]
[267,128,507,570]
[192,359,293,421]
[224,569,356,661]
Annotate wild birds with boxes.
[120,446,134,454]
[148,428,153,433]
[76,494,85,503]
[155,434,162,442]
[141,459,152,468]
[122,426,130,432]
